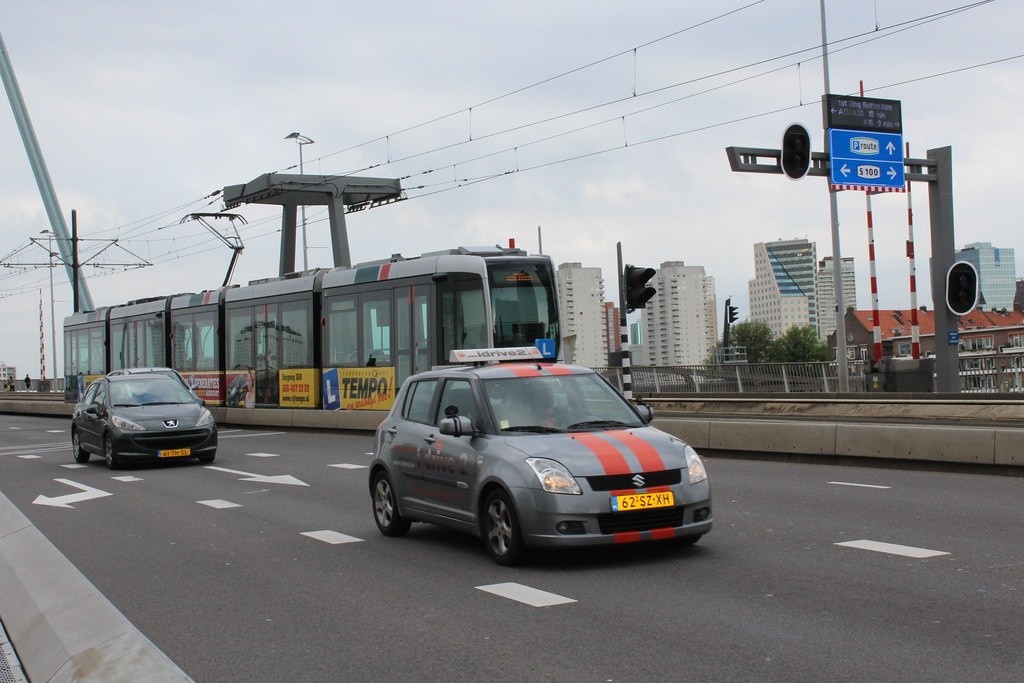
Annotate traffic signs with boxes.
[829,128,904,163]
[830,156,906,188]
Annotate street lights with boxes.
[284,132,316,267]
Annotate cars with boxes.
[71,372,218,471]
[103,366,200,399]
[368,346,715,568]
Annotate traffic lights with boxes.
[729,306,739,323]
[624,264,657,309]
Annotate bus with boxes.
[63,234,563,433]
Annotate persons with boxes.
[245,373,255,408]
[25,374,31,390]
[527,387,568,427]
[4,376,15,391]
